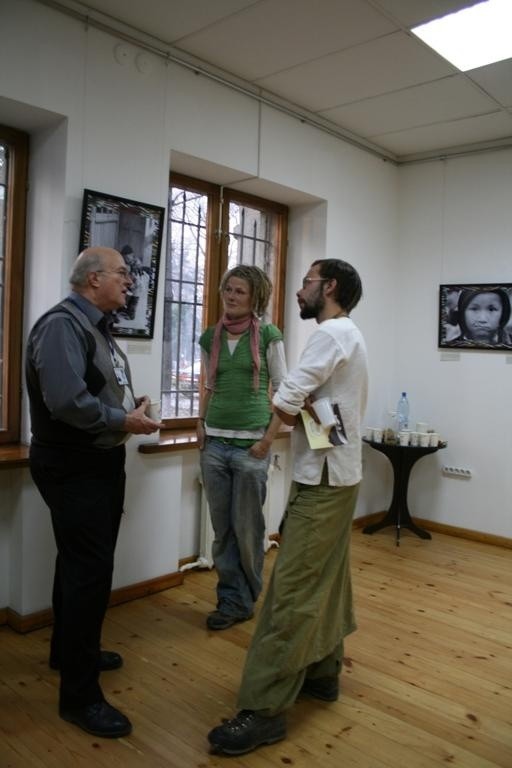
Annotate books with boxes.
[296,403,352,449]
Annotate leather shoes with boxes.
[56,674,134,741]
[50,645,125,672]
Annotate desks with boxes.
[359,433,443,545]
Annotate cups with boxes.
[148,399,162,425]
[365,424,441,446]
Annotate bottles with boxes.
[394,392,411,433]
[383,411,398,445]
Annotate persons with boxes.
[448,286,511,344]
[25,245,167,737]
[208,257,368,756]
[198,264,287,631]
[121,244,149,275]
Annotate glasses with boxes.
[91,266,138,280]
[301,274,336,286]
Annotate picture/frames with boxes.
[76,186,166,340]
[437,281,512,353]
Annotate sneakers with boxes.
[301,676,339,705]
[206,702,290,755]
[202,599,256,631]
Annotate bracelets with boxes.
[198,415,206,421]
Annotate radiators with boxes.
[178,462,281,573]
